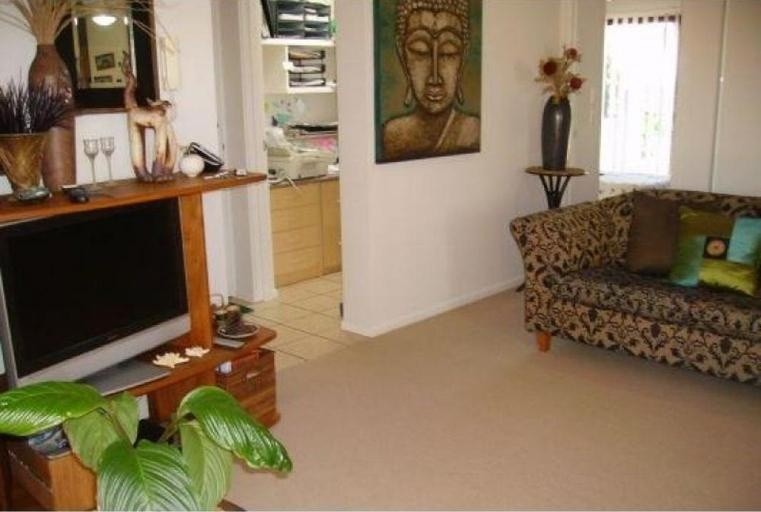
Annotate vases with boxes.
[540,95,570,171]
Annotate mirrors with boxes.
[54,0,160,117]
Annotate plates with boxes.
[218,323,259,338]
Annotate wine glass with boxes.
[84,139,101,193]
[100,137,119,188]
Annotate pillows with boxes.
[624,189,720,277]
[657,204,761,304]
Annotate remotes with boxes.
[214,337,245,350]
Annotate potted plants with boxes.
[0,0,181,195]
[0,67,82,202]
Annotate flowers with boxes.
[533,43,586,97]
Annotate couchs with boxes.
[507,187,761,387]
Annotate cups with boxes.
[213,309,228,329]
[227,305,241,328]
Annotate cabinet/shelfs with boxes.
[319,177,339,275]
[270,182,326,290]
[0,172,283,512]
[259,0,337,139]
[515,166,587,292]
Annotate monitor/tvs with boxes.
[0,196,194,398]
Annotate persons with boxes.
[384,0,480,152]
